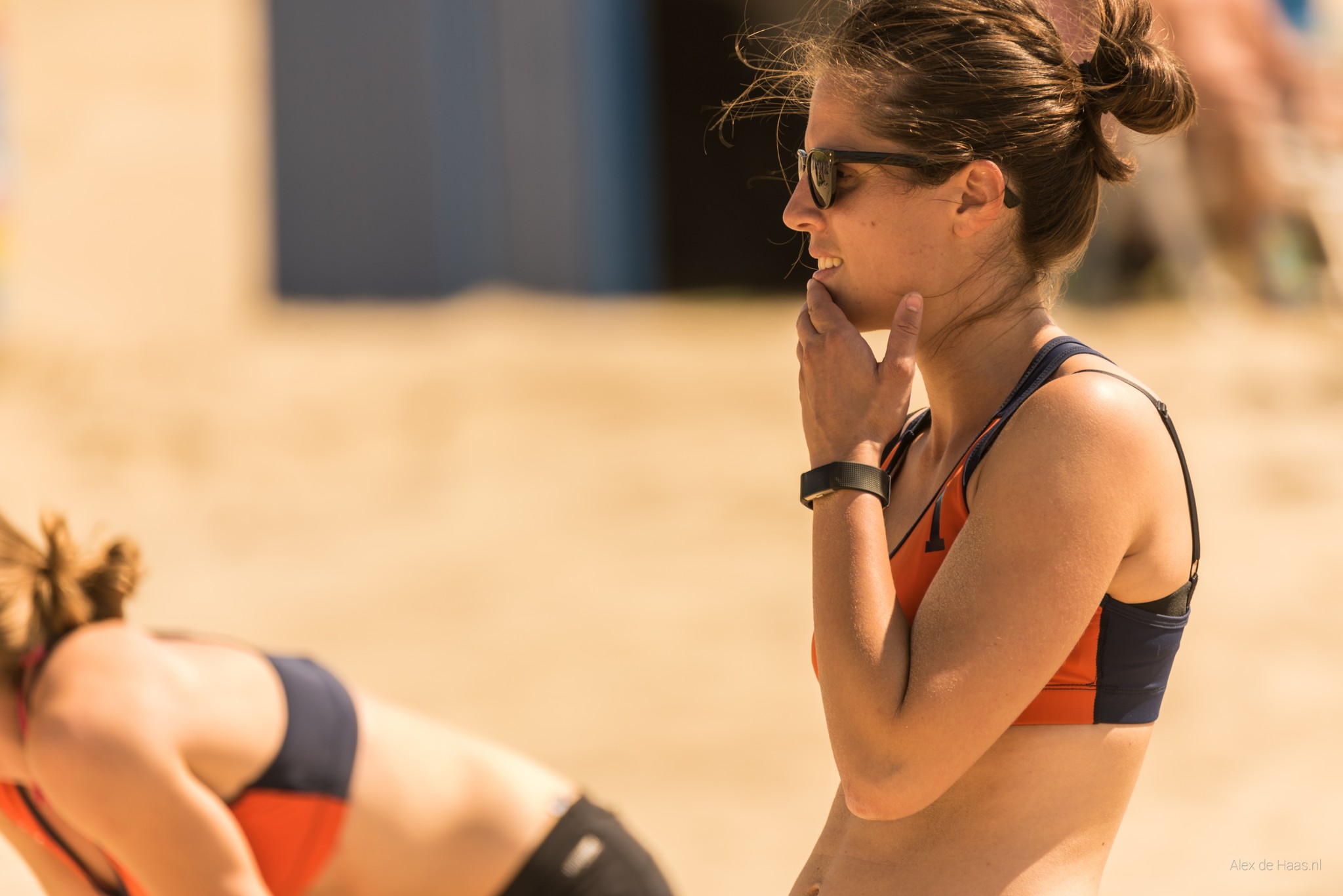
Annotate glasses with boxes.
[796,147,1022,209]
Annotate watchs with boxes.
[798,462,888,504]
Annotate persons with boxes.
[714,0,1203,896]
[0,521,668,896]
